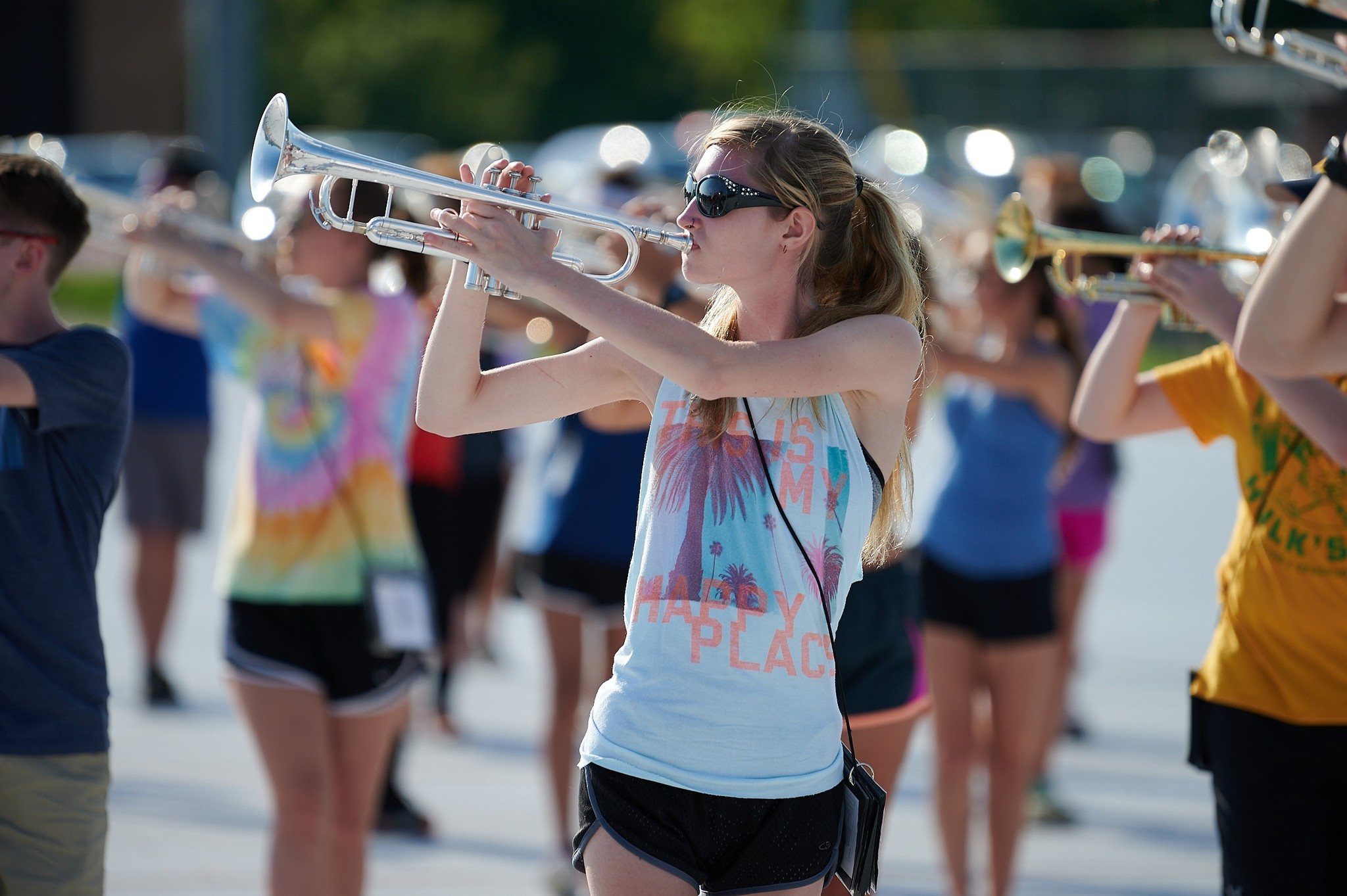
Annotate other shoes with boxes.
[372,777,426,834]
[145,664,175,705]
[1019,779,1072,826]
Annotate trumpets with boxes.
[1209,0,1347,90]
[21,129,275,265]
[248,91,695,301]
[992,190,1270,335]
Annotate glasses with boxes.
[683,171,829,231]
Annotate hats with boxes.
[1266,173,1325,206]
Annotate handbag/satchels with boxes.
[362,563,434,655]
[835,741,887,896]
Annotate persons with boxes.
[1067,144,1347,896]
[116,154,1118,896]
[0,154,130,896]
[414,113,922,896]
